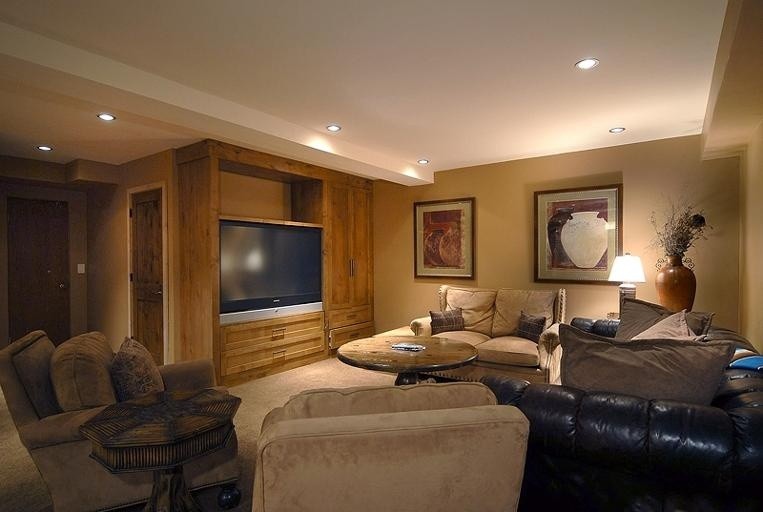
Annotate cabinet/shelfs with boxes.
[216,309,330,390]
[288,177,376,359]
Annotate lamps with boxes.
[687,212,705,228]
[606,251,646,319]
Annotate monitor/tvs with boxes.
[219,220,323,326]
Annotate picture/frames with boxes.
[531,182,625,288]
[411,196,477,281]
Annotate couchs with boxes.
[392,311,760,512]
[248,377,533,512]
[409,285,570,388]
[1,321,248,512]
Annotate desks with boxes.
[81,383,246,510]
[335,332,481,386]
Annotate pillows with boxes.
[610,290,716,343]
[627,308,696,340]
[428,306,466,336]
[515,309,547,344]
[112,340,167,399]
[552,318,737,407]
[48,329,115,408]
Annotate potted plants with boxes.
[646,199,713,315]
